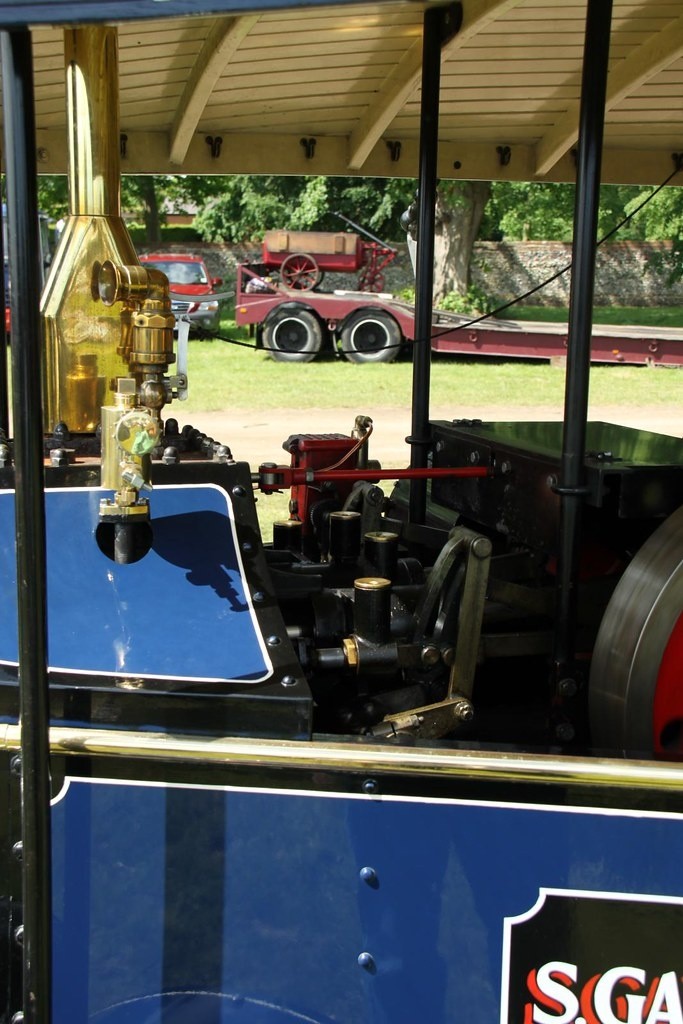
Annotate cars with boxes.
[136,252,220,338]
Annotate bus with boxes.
[1,200,59,341]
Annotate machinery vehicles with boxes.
[233,230,683,370]
